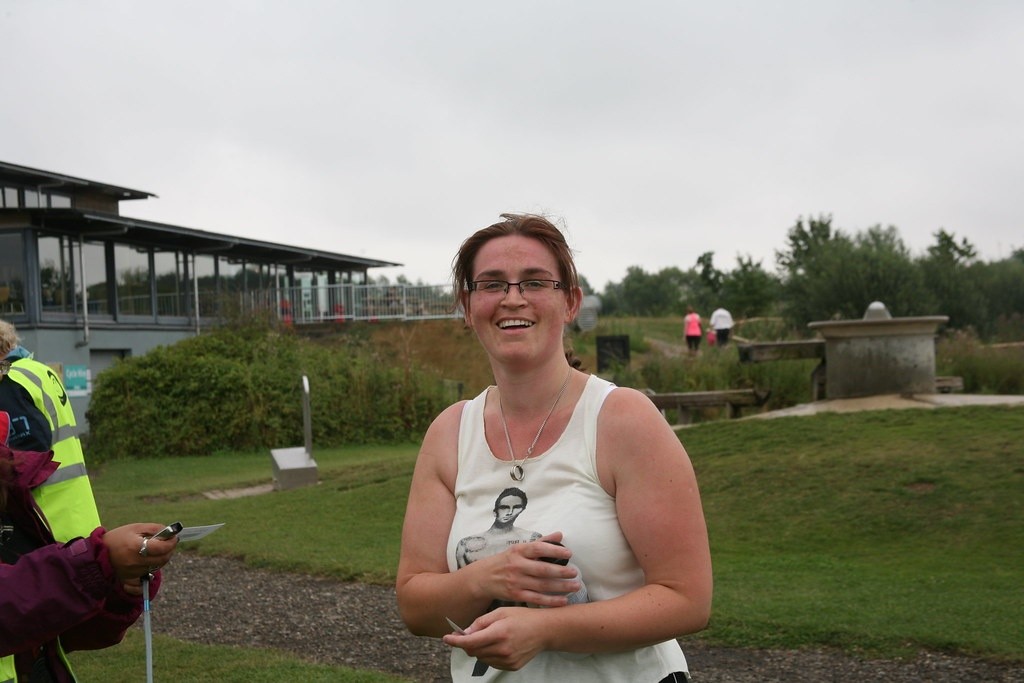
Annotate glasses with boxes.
[464,279,568,293]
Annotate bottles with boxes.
[538,540,593,660]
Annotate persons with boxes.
[683,304,700,359]
[391,212,715,682]
[706,303,733,349]
[1,315,180,683]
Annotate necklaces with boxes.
[494,365,574,482]
